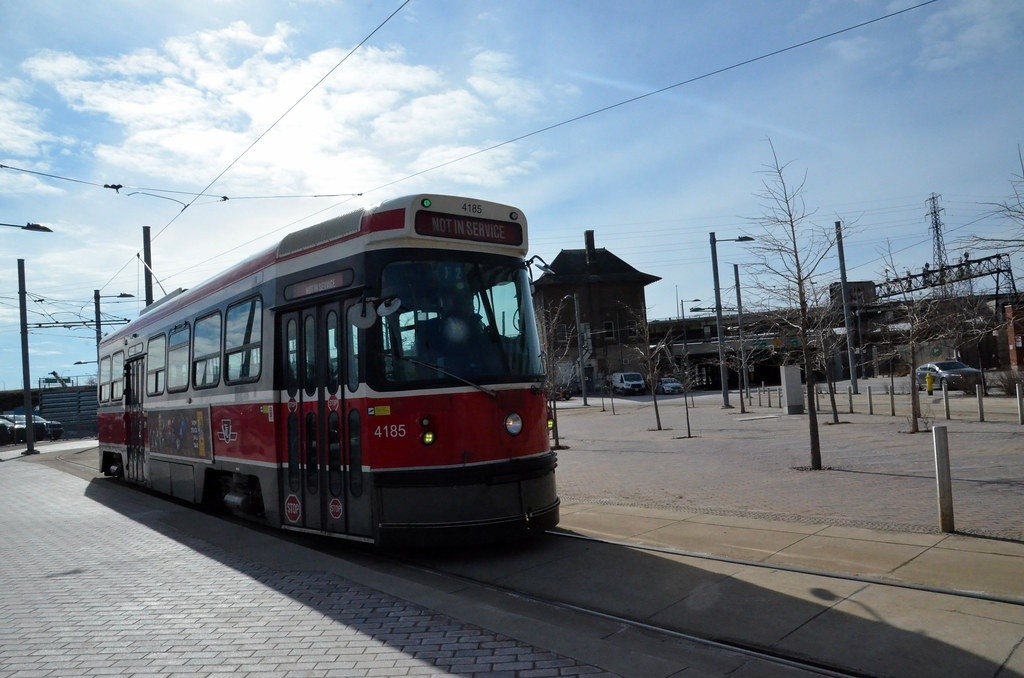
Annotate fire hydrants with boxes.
[925,371,934,396]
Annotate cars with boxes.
[913,361,987,395]
[656,377,683,394]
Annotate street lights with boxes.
[681,299,701,391]
[710,232,755,408]
[93,289,135,365]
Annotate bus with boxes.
[98,194,560,554]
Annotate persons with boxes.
[152,414,186,448]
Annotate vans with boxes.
[612,372,646,396]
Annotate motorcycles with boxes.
[550,386,572,402]
